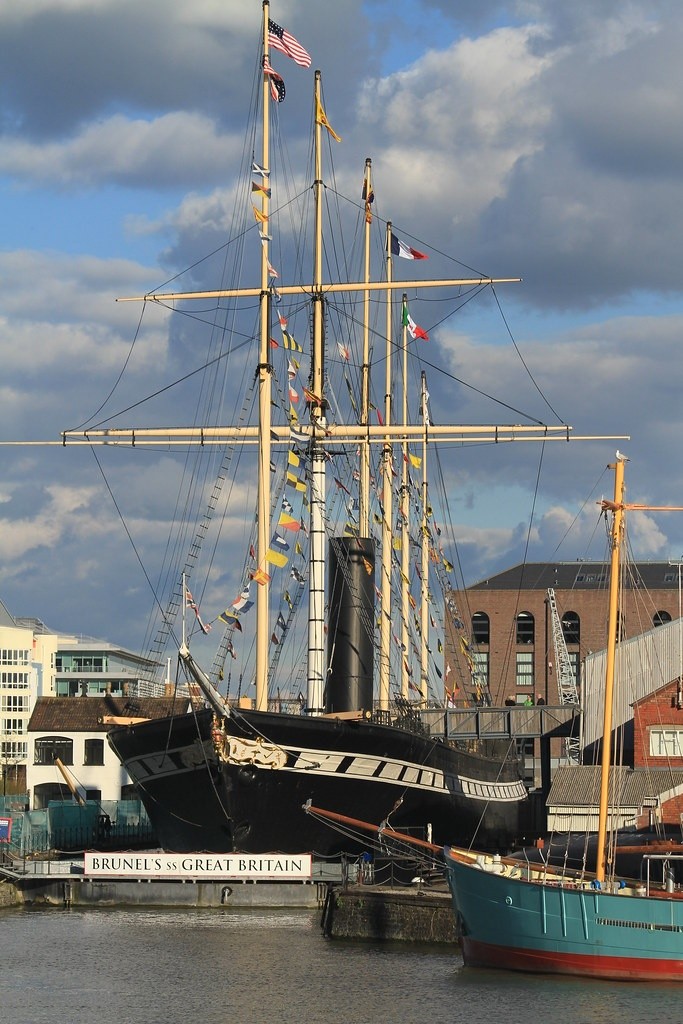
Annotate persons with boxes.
[505,695,516,706]
[537,694,544,705]
[523,695,533,706]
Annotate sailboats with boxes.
[441,450,683,983]
[68,11,629,882]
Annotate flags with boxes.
[362,179,374,202]
[314,98,340,142]
[259,230,271,240]
[334,401,484,708]
[205,533,305,698]
[268,17,311,68]
[402,306,429,341]
[270,338,279,348]
[386,233,428,260]
[268,262,279,278]
[253,164,270,178]
[278,422,331,539]
[186,588,206,635]
[274,288,302,352]
[304,389,321,405]
[338,343,350,360]
[288,358,300,425]
[253,206,269,221]
[252,182,271,199]
[265,57,285,102]
